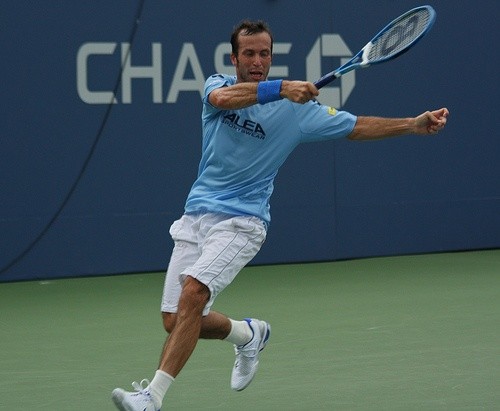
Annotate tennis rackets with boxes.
[312,4,438,90]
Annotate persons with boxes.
[111,22,449,411]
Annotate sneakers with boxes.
[231,319,271,391]
[112,378,162,411]
